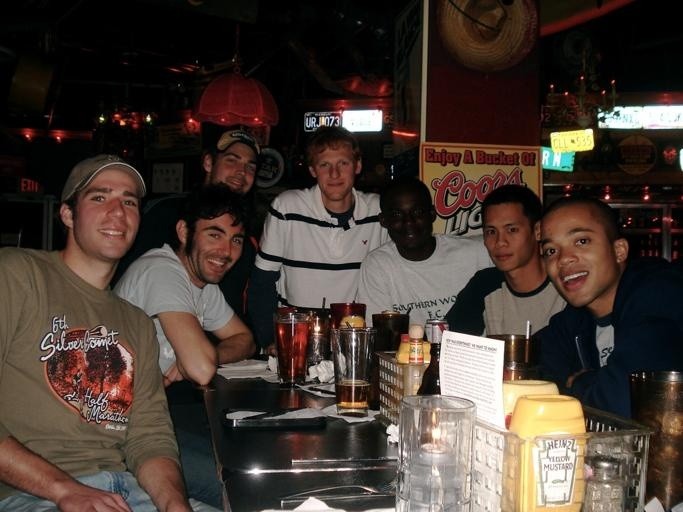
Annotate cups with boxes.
[489,333,527,383]
[271,313,312,389]
[329,302,366,330]
[370,313,409,354]
[393,393,475,512]
[626,367,683,512]
[328,326,378,419]
[299,307,332,375]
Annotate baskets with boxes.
[475,406,660,512]
[375,350,431,425]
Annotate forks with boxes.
[279,473,402,503]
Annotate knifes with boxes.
[291,454,399,464]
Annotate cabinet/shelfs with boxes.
[604,203,682,264]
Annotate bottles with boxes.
[402,323,442,396]
[582,454,625,512]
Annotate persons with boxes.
[239,125,394,362]
[439,182,570,343]
[0,150,227,512]
[525,190,682,424]
[355,173,499,353]
[117,126,280,361]
[105,181,259,510]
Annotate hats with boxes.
[216,130,261,155]
[61,154,146,202]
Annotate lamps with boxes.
[193,19,281,127]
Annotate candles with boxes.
[421,426,451,468]
